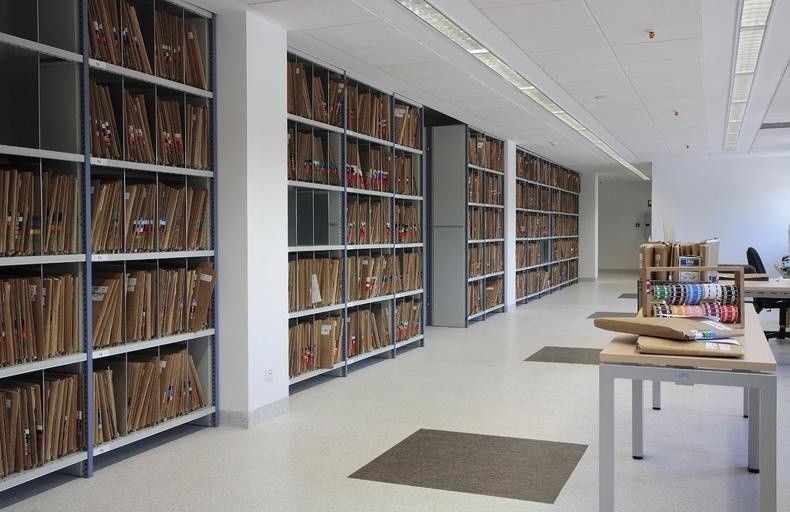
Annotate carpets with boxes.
[347,427,589,504]
[586,292,638,319]
[524,345,603,366]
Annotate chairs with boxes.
[746,246,790,340]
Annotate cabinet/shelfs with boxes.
[429,123,584,329]
[2,1,222,491]
[285,49,428,397]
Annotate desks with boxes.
[597,279,790,511]
